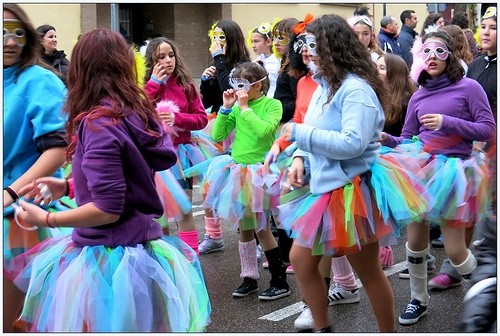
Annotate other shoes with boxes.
[431,233,444,247]
[427,259,464,290]
[285,264,295,274]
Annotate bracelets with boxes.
[435,114,442,131]
[201,76,208,81]
[46,212,58,228]
[151,75,162,85]
[219,106,232,114]
[64,177,75,200]
[4,186,19,203]
[291,123,296,142]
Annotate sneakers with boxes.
[326,282,360,305]
[255,245,263,263]
[398,255,436,279]
[398,298,428,325]
[257,285,291,300]
[232,277,259,297]
[293,304,315,331]
[196,233,226,255]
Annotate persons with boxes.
[35,24,70,88]
[211,59,291,300]
[370,29,497,324]
[248,5,497,305]
[280,13,396,333]
[195,19,256,253]
[2,3,70,333]
[142,37,209,265]
[10,28,212,333]
